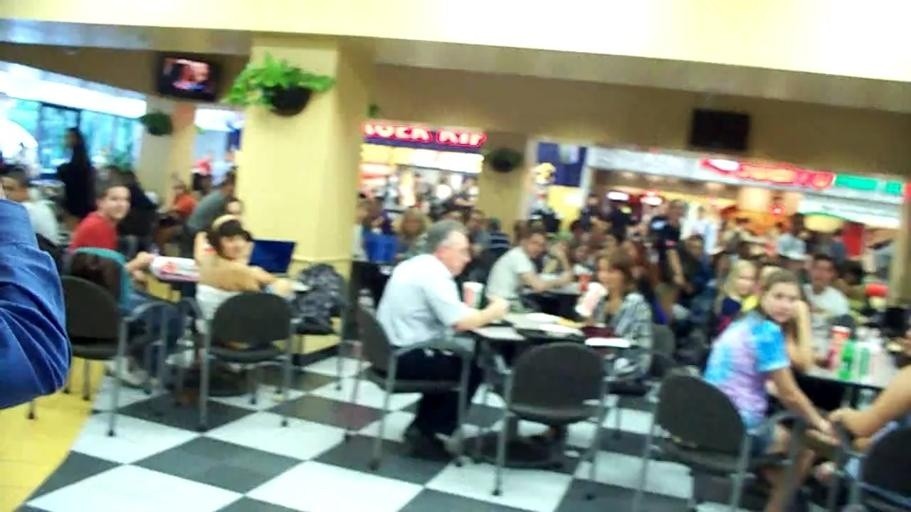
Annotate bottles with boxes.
[859,346,873,376]
[836,340,854,380]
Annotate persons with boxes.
[352,171,909,512]
[2,126,291,410]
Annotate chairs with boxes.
[353,261,910,510]
[25,234,347,438]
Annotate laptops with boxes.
[247,240,296,277]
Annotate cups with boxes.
[576,282,606,316]
[461,282,486,309]
[827,324,850,360]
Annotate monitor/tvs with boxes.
[156,54,220,102]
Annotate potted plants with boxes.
[212,49,336,118]
[483,143,523,174]
